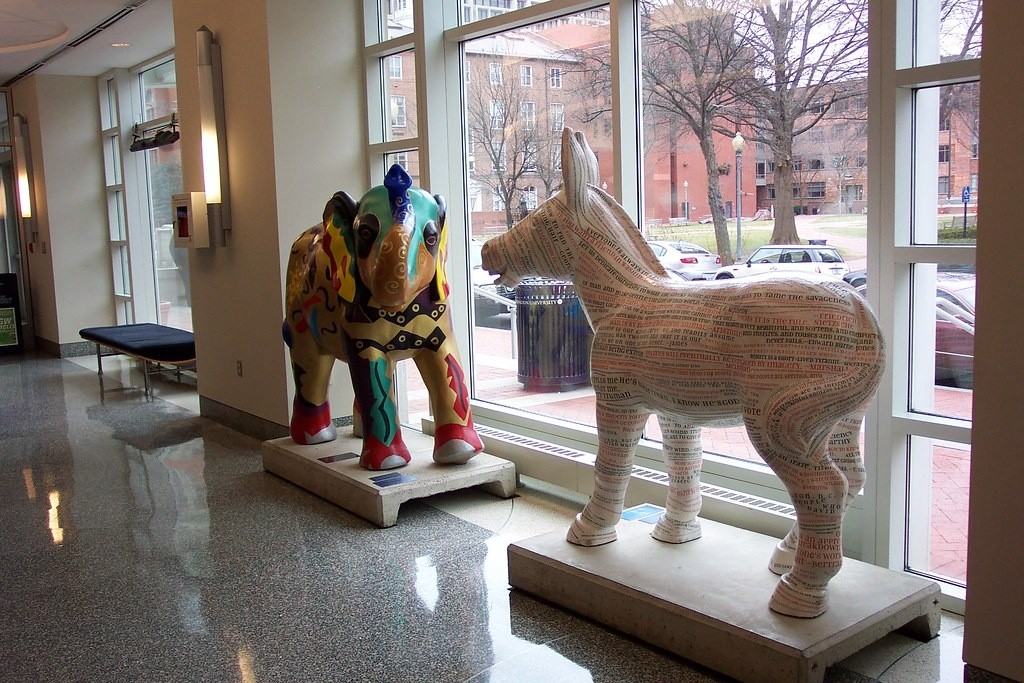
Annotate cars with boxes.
[712,245,849,280]
[473,238,543,317]
[843,260,976,289]
[698,214,713,225]
[647,239,723,279]
[855,271,976,388]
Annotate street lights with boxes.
[683,181,688,221]
[731,132,746,259]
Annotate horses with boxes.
[481,128,886,618]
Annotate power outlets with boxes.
[237,360,242,377]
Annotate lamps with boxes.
[13,112,39,244]
[196,26,231,248]
[130,113,182,153]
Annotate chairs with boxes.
[779,252,792,262]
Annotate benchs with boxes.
[78,323,194,399]
